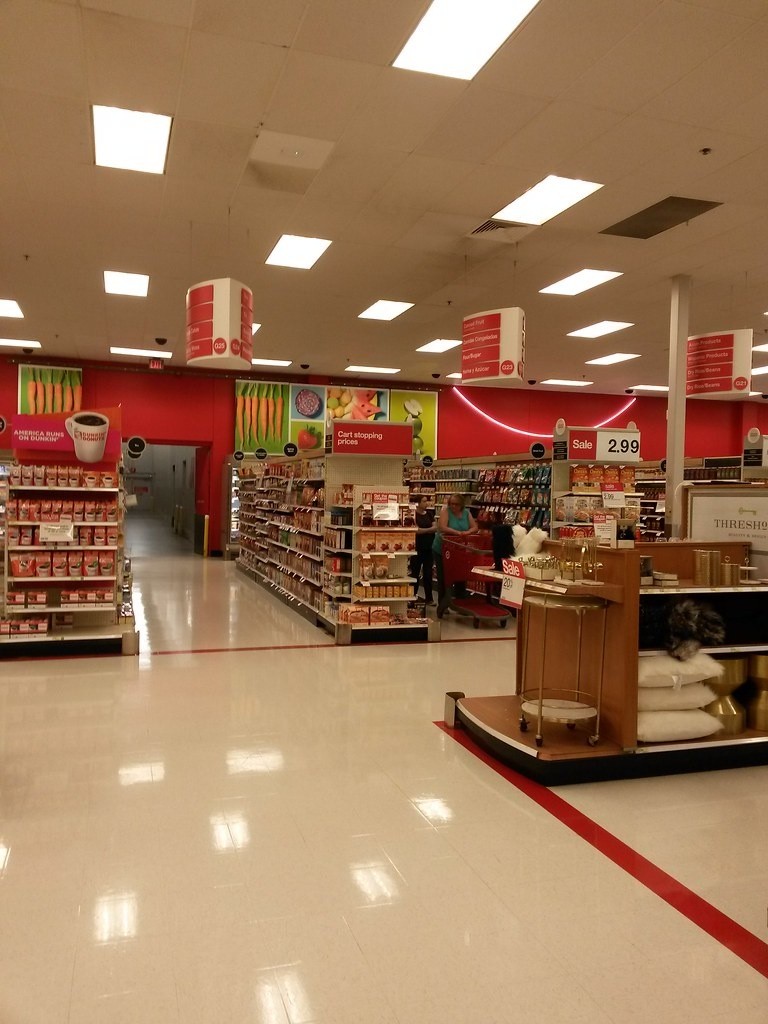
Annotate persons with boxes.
[408,495,437,608]
[432,493,478,618]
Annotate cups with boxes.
[65,411,109,463]
[693,550,722,586]
[12,476,114,576]
[721,563,739,586]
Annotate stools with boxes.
[523,594,608,747]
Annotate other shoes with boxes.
[437,606,444,619]
[425,600,437,606]
[444,608,450,615]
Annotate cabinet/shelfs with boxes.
[0,473,123,642]
[236,451,768,762]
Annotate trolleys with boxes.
[437,532,512,629]
